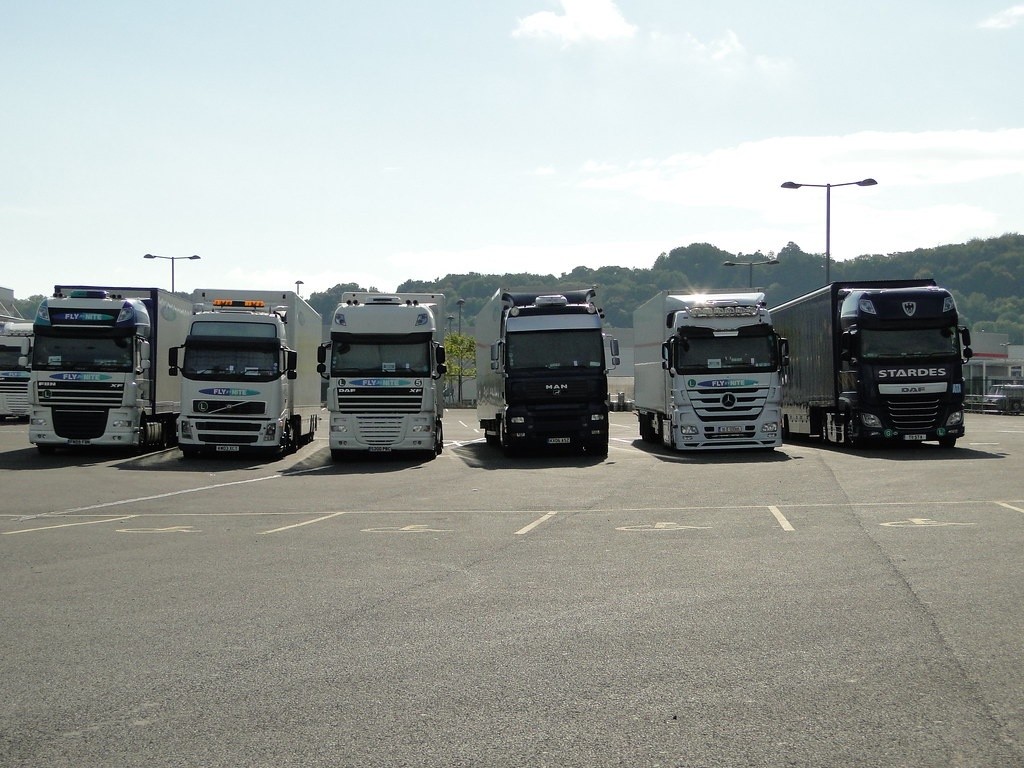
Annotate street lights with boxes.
[457,299,465,402]
[722,260,780,290]
[144,254,202,293]
[295,281,305,294]
[999,342,1011,377]
[780,179,878,286]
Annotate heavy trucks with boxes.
[633,291,790,454]
[169,289,324,462]
[318,291,448,462]
[476,286,621,458]
[0,321,35,424]
[767,278,974,451]
[19,285,195,458]
